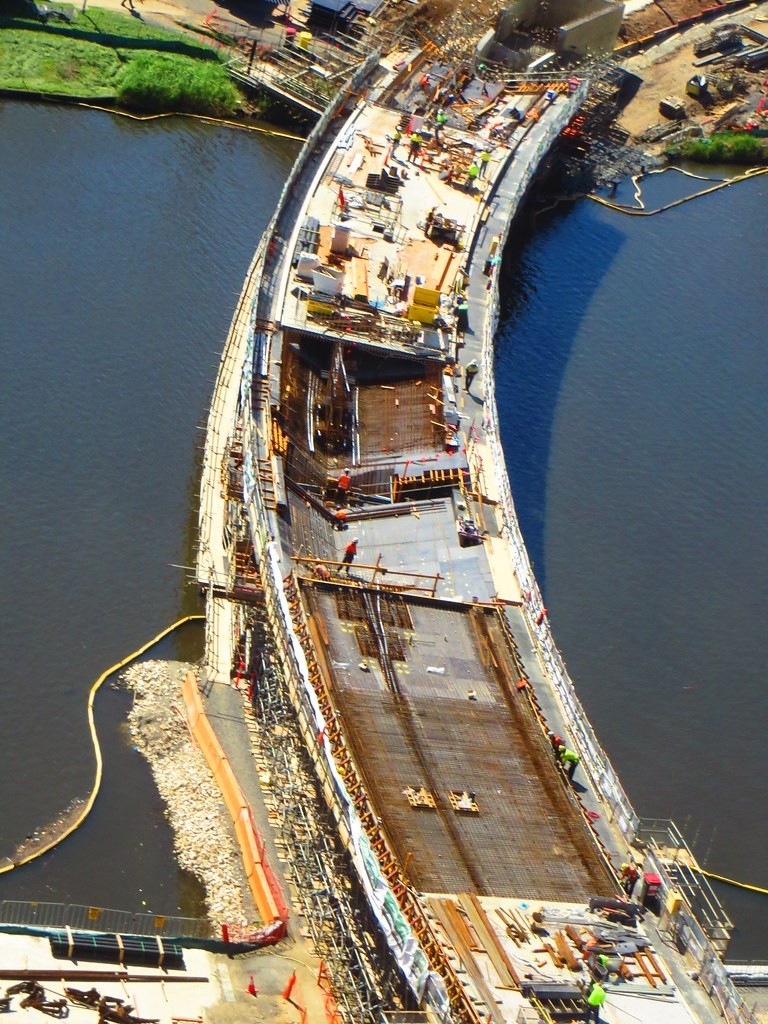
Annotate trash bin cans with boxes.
[636,872,661,906]
[457,304,469,328]
[482,257,493,276]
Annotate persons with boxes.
[467,159,479,188]
[315,557,331,580]
[122,0,134,9]
[617,863,638,895]
[407,129,420,163]
[436,109,446,130]
[584,982,604,1024]
[337,466,351,499]
[332,504,348,531]
[390,126,402,158]
[547,730,580,783]
[335,537,358,577]
[462,358,479,395]
[478,146,491,178]
[421,74,431,91]
[490,121,507,138]
[424,206,436,237]
